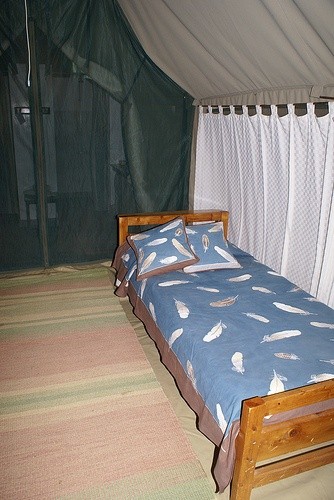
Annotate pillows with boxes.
[182,220,241,274]
[126,216,201,281]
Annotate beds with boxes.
[111,210,334,500]
